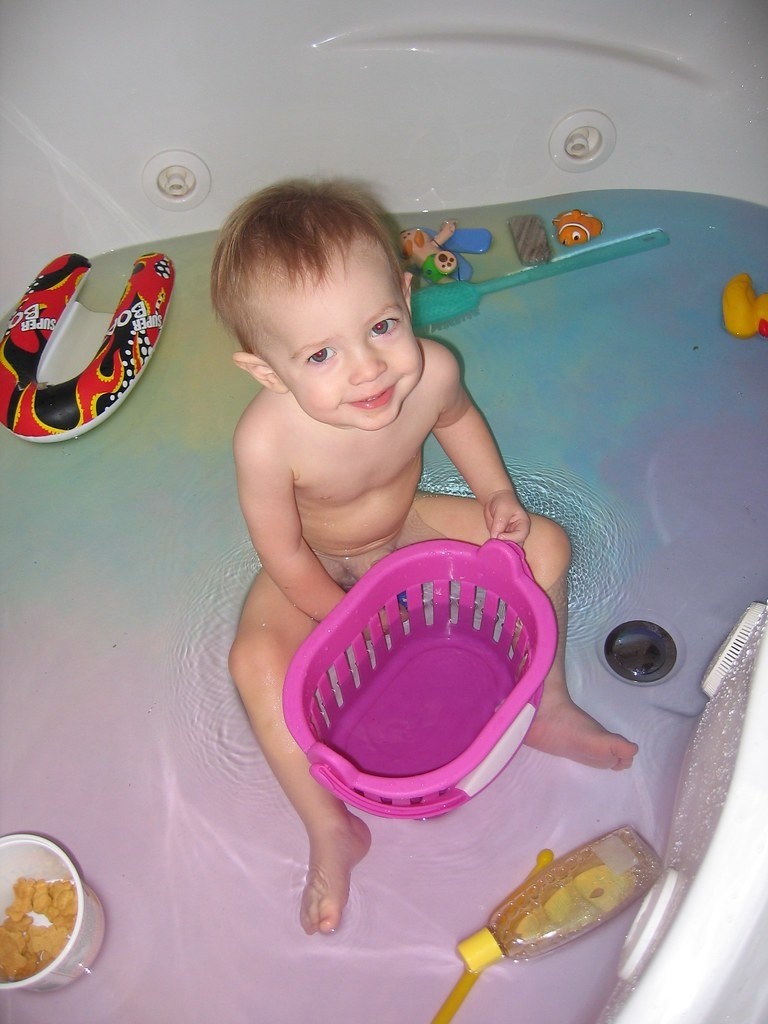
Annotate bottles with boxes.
[455,826,664,973]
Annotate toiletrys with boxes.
[455,824,665,974]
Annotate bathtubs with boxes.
[0,0,767,1023]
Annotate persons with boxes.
[212,181,639,935]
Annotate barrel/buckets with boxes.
[0,832,105,993]
